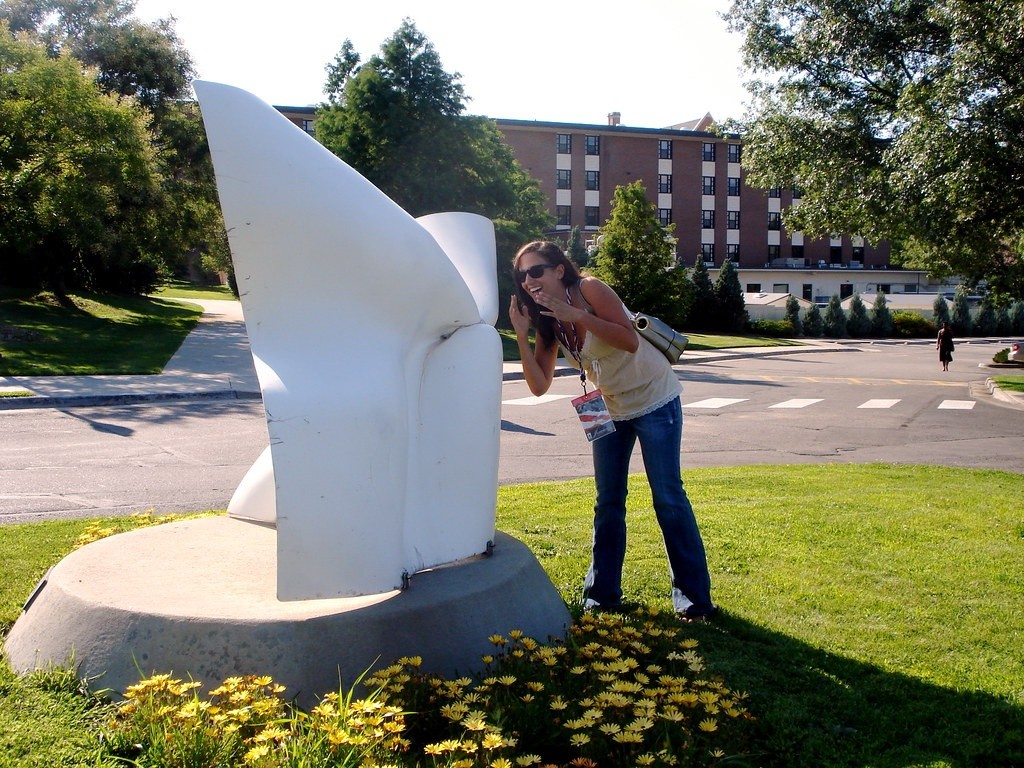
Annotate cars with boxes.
[1007,342,1024,363]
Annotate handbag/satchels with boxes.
[574,278,689,364]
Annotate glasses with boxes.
[516,264,555,282]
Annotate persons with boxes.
[936,321,954,372]
[509,239,720,623]
